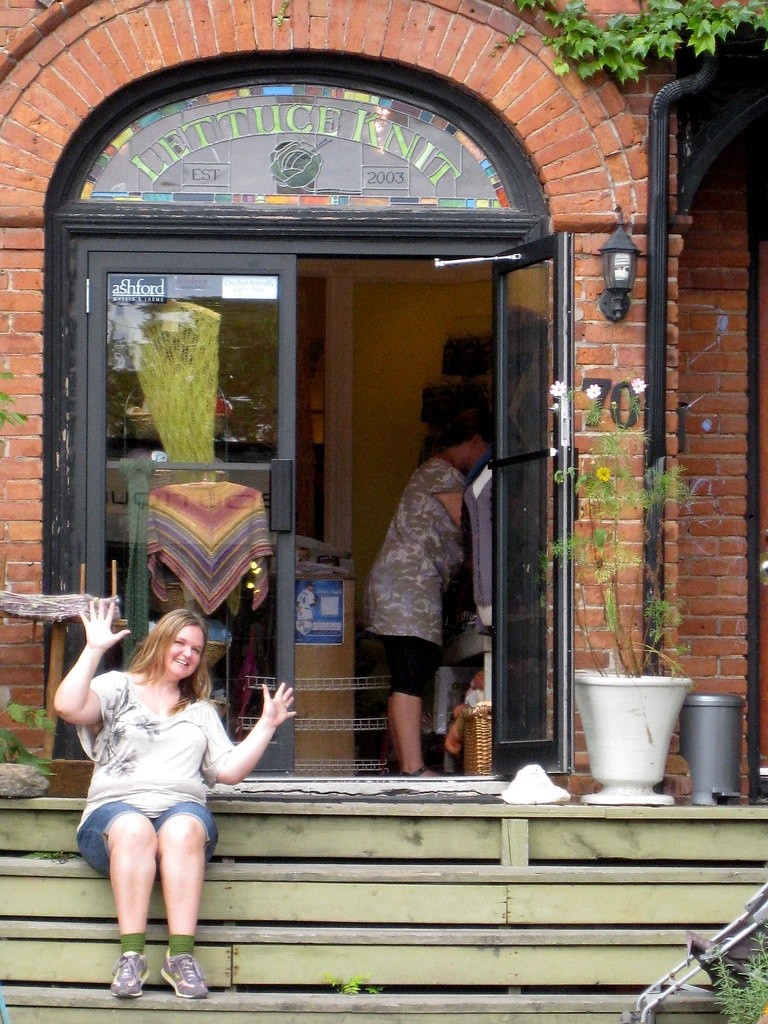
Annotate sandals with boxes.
[401,766,442,777]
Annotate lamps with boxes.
[596,206,643,324]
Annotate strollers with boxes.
[615,882,767,1024]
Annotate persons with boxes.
[295,582,320,623]
[361,424,499,779]
[53,599,297,999]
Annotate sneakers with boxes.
[111,951,150,998]
[161,948,209,999]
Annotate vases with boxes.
[567,670,696,806]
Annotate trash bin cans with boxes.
[680,693,744,806]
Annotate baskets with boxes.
[151,582,208,618]
[204,640,227,668]
[462,701,493,776]
[211,700,230,721]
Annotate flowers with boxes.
[538,378,697,690]
